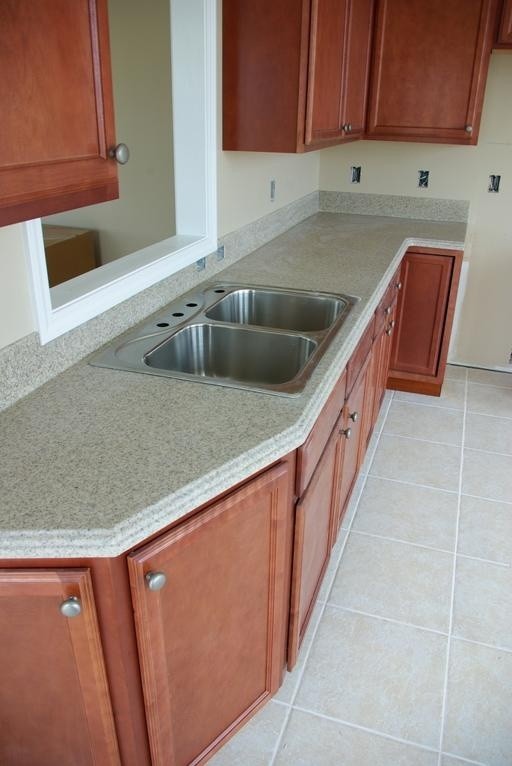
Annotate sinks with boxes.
[178,281,357,336]
[87,323,324,400]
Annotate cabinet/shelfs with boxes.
[125,458,295,766]
[0,566,123,766]
[287,243,402,674]
[221,0,497,154]
[388,251,464,396]
[496,0,511,51]
[0,0,132,228]
